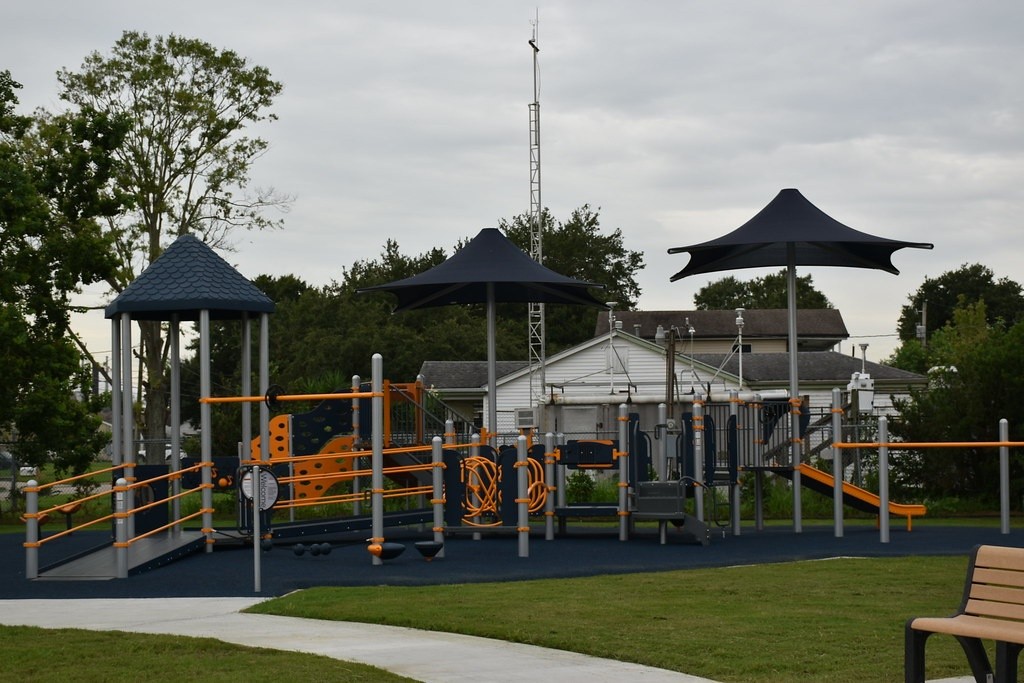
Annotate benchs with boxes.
[903,542,1024,683]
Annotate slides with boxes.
[801,461,927,519]
